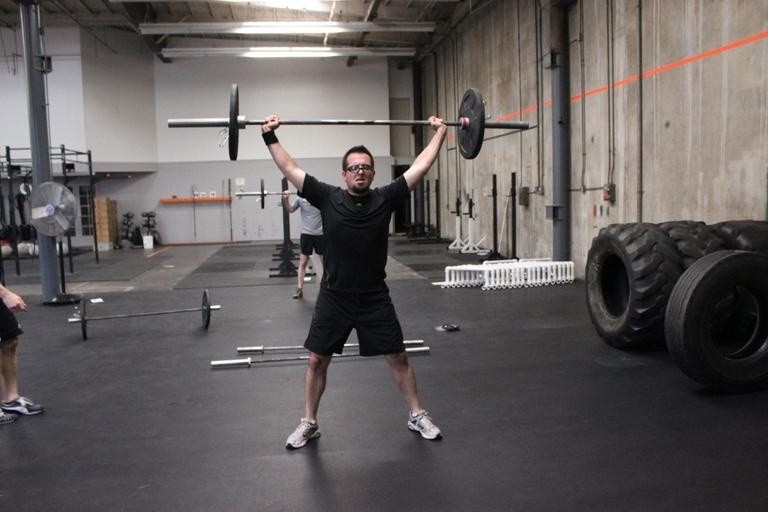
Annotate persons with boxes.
[259,113,448,453]
[0,283,45,426]
[281,189,325,299]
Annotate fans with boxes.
[26,181,84,305]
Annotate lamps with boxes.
[139,22,438,35]
[161,47,416,57]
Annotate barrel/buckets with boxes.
[143,236,153,249]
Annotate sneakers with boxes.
[285,418,319,448]
[410,411,443,440]
[0,397,43,424]
[292,290,303,299]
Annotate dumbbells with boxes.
[121,210,157,241]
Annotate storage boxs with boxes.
[91,196,118,251]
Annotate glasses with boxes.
[345,165,375,173]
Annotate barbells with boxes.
[68,287,221,341]
[237,179,300,209]
[168,85,530,160]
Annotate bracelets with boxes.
[262,129,280,145]
